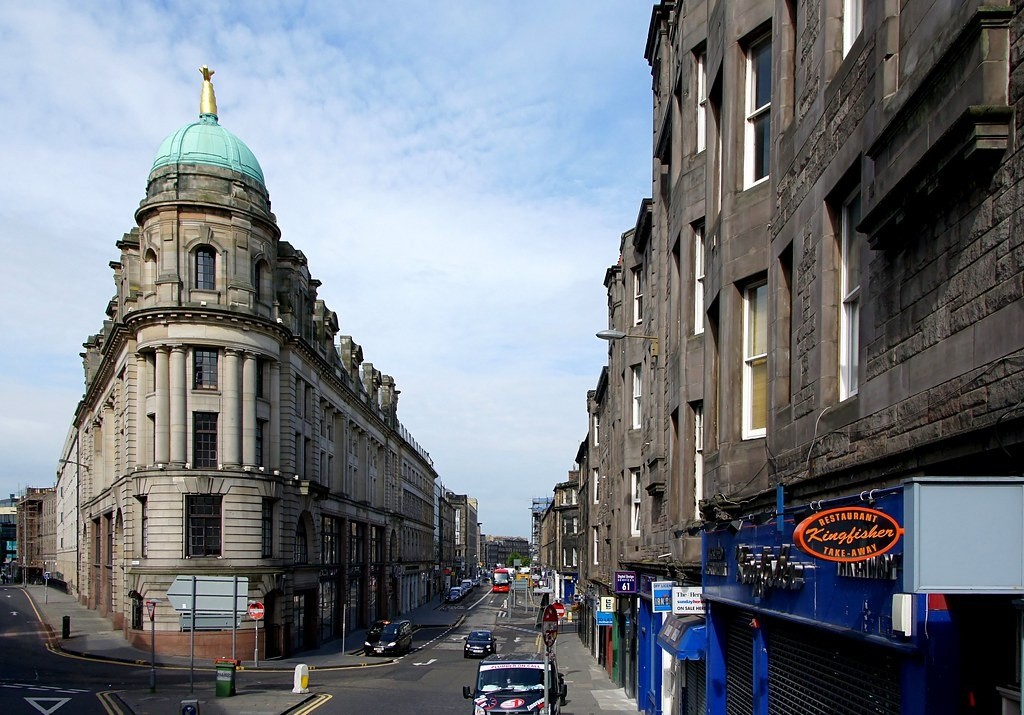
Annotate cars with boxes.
[447,579,480,603]
[463,630,497,658]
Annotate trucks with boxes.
[463,653,567,715]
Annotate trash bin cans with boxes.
[62,616,71,640]
[214,658,240,697]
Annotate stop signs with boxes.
[543,605,558,646]
[249,603,264,620]
[553,603,565,619]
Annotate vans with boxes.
[364,619,413,657]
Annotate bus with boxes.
[492,569,510,593]
